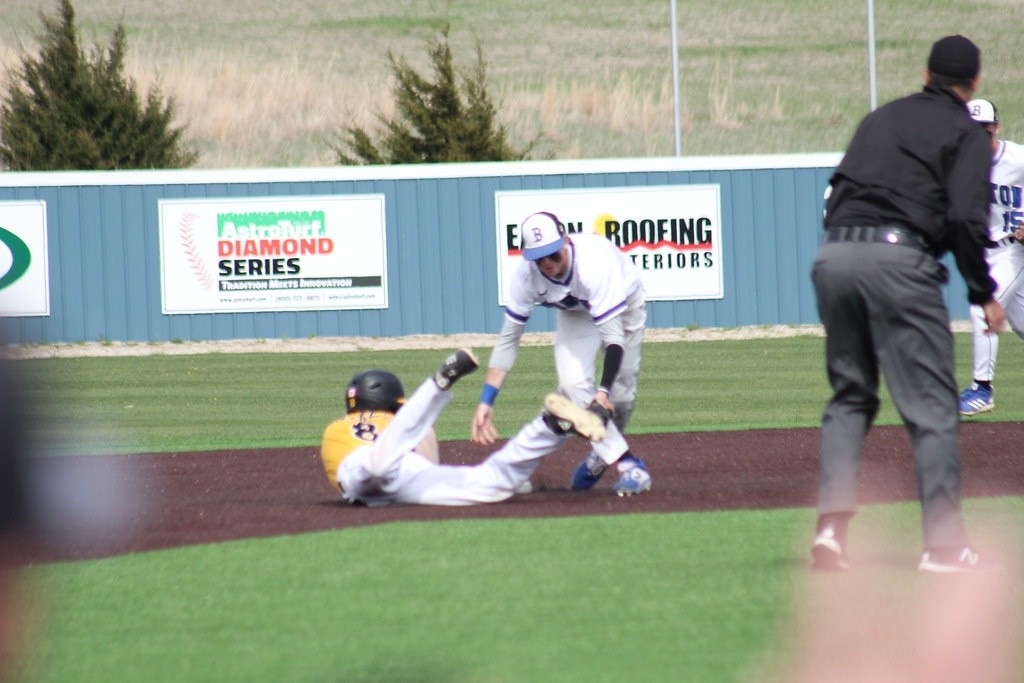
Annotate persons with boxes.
[956,100,1024,415]
[809,35,1006,575]
[472,212,652,496]
[321,348,605,509]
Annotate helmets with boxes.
[345,370,404,414]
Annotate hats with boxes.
[518,212,565,261]
[966,99,998,123]
[928,33,980,81]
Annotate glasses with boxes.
[535,249,562,264]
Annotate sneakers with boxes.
[434,347,480,391]
[811,516,852,573]
[571,450,609,490]
[542,393,608,443]
[958,380,995,416]
[918,545,989,577]
[611,456,652,497]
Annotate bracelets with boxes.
[598,387,608,393]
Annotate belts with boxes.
[987,236,1014,248]
[827,226,928,252]
